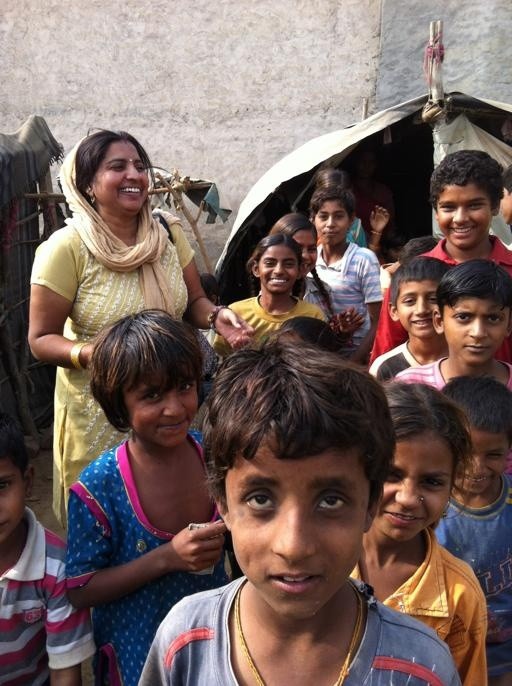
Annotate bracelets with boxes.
[207,302,234,333]
[370,229,382,235]
[71,341,91,373]
[369,243,380,251]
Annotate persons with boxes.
[351,380,487,685]
[383,258,512,394]
[316,169,389,254]
[498,164,512,251]
[309,184,383,362]
[138,329,462,686]
[1,421,98,686]
[370,150,512,370]
[270,213,367,346]
[63,308,231,686]
[29,126,253,532]
[369,256,454,384]
[207,232,329,357]
[435,376,512,685]
